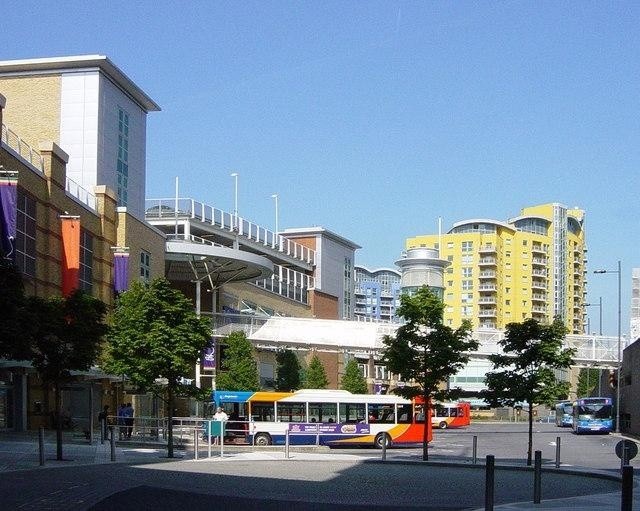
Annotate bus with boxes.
[202,390,359,444]
[379,400,470,430]
[572,396,613,435]
[245,389,433,449]
[555,402,573,428]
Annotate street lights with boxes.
[594,259,623,434]
[582,297,602,397]
[231,172,239,229]
[271,193,279,246]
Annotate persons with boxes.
[103,402,134,440]
[212,406,228,422]
